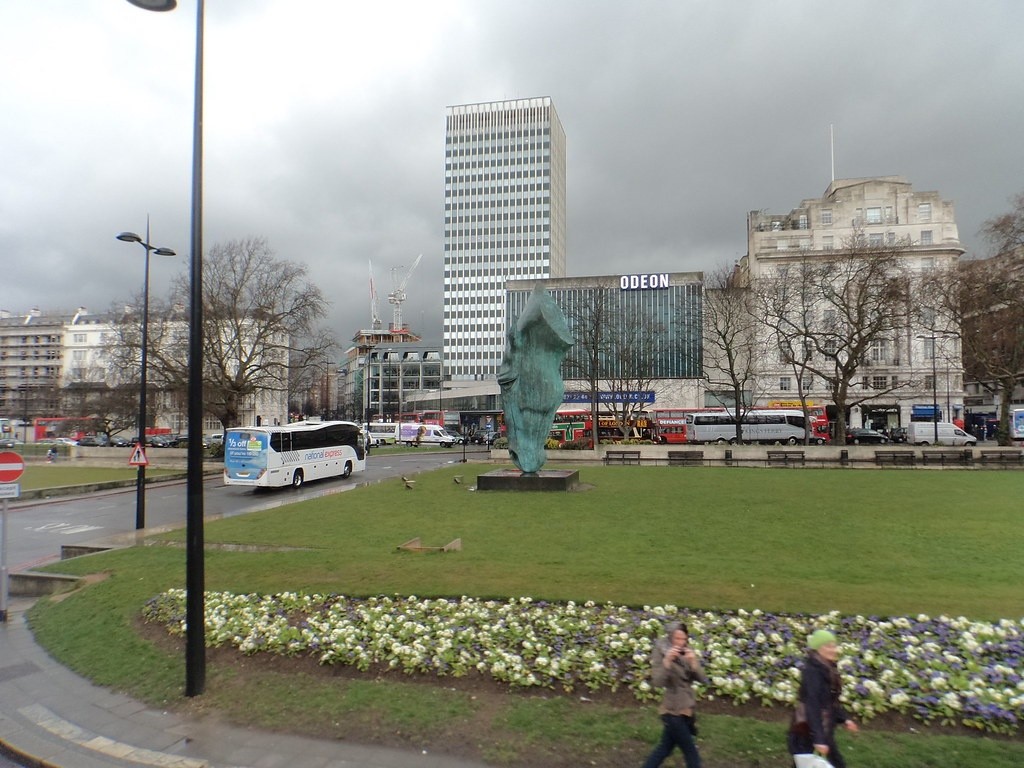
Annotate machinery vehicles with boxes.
[387,255,427,341]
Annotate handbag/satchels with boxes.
[792,750,834,768]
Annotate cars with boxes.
[79,436,107,446]
[470,429,500,445]
[0,439,25,448]
[445,430,465,445]
[36,439,55,447]
[890,427,908,444]
[56,437,80,446]
[845,428,894,445]
[109,433,189,447]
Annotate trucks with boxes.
[908,422,978,447]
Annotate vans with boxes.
[396,423,457,448]
[210,433,224,445]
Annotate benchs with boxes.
[664,451,704,467]
[766,451,806,466]
[602,450,640,465]
[874,451,916,466]
[980,450,1024,466]
[921,450,966,465]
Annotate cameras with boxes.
[678,649,685,655]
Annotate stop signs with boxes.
[0,452,26,483]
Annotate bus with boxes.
[496,409,596,450]
[598,416,661,444]
[361,422,397,445]
[687,408,813,445]
[33,415,98,444]
[0,417,12,440]
[654,403,831,445]
[223,416,367,490]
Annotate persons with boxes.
[890,426,894,434]
[47,443,59,465]
[787,630,851,768]
[884,425,889,438]
[640,620,708,768]
[467,429,473,443]
[412,426,427,442]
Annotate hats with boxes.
[807,629,836,650]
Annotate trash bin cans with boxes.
[725,450,733,465]
[840,450,849,465]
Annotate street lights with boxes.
[917,331,951,447]
[320,355,336,422]
[113,209,178,531]
[124,0,219,699]
